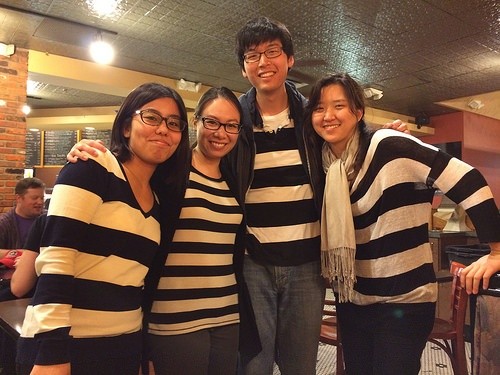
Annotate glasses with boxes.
[196,114,245,135]
[131,108,186,133]
[241,44,288,63]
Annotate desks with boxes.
[469,272,500,375]
[0,298,31,342]
[430,229,472,322]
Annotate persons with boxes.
[0,17,500,375]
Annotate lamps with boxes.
[88,29,114,65]
[468,99,485,110]
[363,88,384,100]
[0,42,15,58]
[176,78,202,93]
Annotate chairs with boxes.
[319,278,346,375]
[427,262,468,375]
